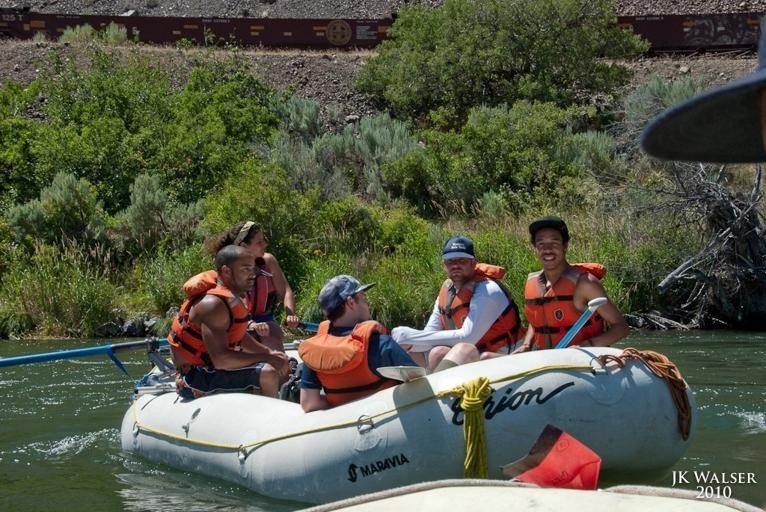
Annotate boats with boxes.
[120,341,698,505]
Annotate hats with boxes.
[318,274,376,316]
[441,236,475,260]
[529,216,568,244]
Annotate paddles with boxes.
[377,366,427,383]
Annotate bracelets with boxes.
[588,339,594,347]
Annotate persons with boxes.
[168,245,290,398]
[392,237,525,374]
[208,218,298,355]
[299,273,481,412]
[509,216,629,353]
